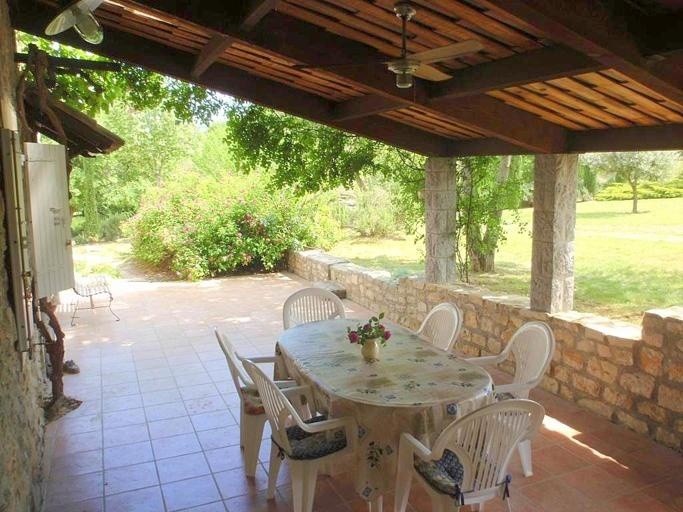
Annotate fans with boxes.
[291,4,485,86]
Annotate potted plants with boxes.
[345,312,393,360]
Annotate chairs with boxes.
[242,358,363,512]
[281,287,347,332]
[395,398,547,512]
[214,326,318,480]
[413,300,463,354]
[465,318,556,476]
[70,267,121,324]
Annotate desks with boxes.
[274,317,498,511]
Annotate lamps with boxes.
[387,60,420,89]
[41,0,107,45]
